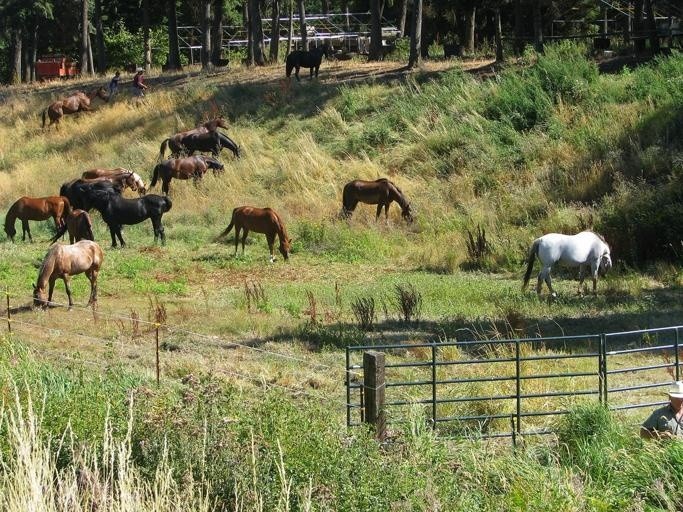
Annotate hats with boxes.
[664,381,683,399]
[135,67,142,71]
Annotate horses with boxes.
[2,167,172,248]
[219,206,292,264]
[286,44,329,82]
[150,155,225,198]
[343,178,413,225]
[154,114,230,164]
[42,92,96,131]
[32,240,104,311]
[181,130,240,160]
[521,229,612,297]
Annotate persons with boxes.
[640,380,683,440]
[132,67,148,97]
[363,33,371,54]
[108,71,121,102]
